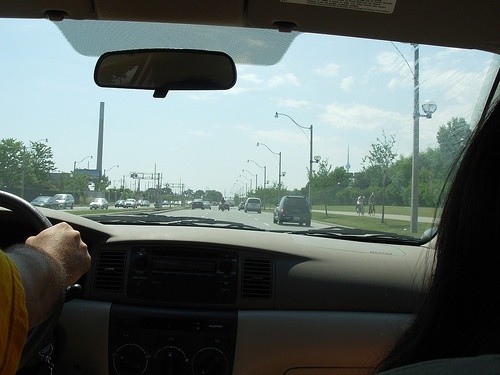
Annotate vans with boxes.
[243,197,262,213]
[54,193,74,209]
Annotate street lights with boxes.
[274,111,321,211]
[21,139,49,199]
[73,156,93,185]
[104,165,120,177]
[406,100,439,234]
[247,160,270,210]
[242,169,258,196]
[256,142,286,204]
[230,175,253,198]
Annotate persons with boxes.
[376,100,500,371]
[356,192,376,213]
[0,221,92,375]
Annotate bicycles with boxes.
[356,202,377,216]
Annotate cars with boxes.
[114,198,150,208]
[238,202,245,211]
[154,200,189,206]
[89,198,109,210]
[192,198,235,211]
[29,196,60,211]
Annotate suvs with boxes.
[273,194,312,226]
